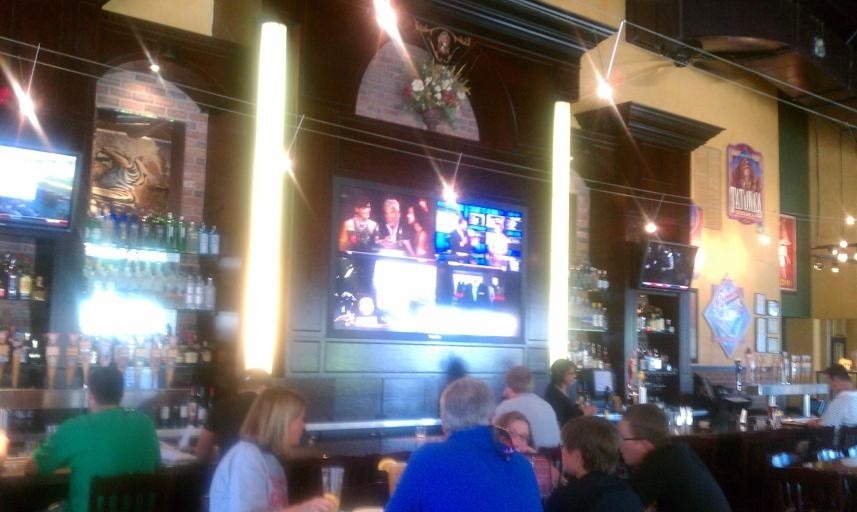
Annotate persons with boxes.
[25,365,161,512]
[338,195,509,267]
[195,372,339,511]
[382,376,543,512]
[494,358,734,512]
[800,364,857,451]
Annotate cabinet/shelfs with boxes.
[637,289,680,397]
[80,240,213,389]
[563,283,609,372]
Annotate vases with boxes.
[423,108,446,134]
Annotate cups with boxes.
[672,404,693,426]
[320,465,343,511]
[44,424,56,441]
[753,394,784,430]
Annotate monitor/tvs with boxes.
[634,236,698,297]
[0,139,84,234]
[330,176,532,345]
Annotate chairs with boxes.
[280,450,407,502]
[89,471,200,510]
[720,422,857,510]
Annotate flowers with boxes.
[401,58,472,127]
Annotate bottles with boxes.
[0,252,46,303]
[582,391,591,406]
[81,205,222,388]
[568,265,611,372]
[779,351,812,384]
[414,426,424,450]
[158,382,217,429]
[735,360,744,385]
[634,301,675,371]
[9,408,37,431]
[0,325,41,364]
